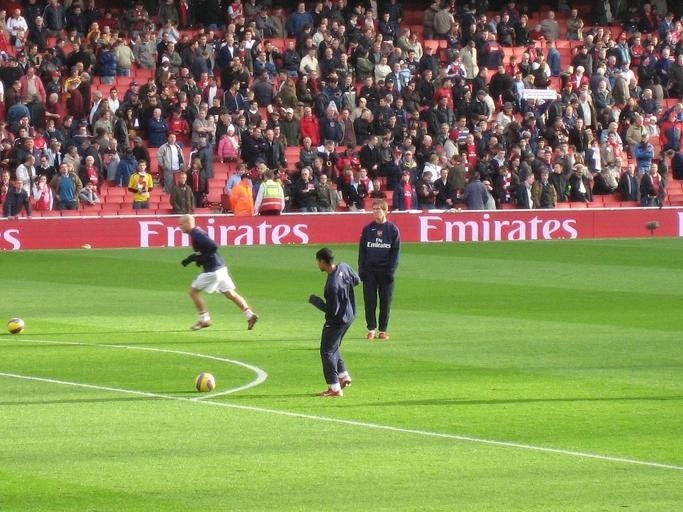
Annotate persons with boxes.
[1,1,682,218]
[358,199,400,342]
[179,213,258,332]
[308,249,360,397]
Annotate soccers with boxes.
[194,373,215,392]
[8,317,24,335]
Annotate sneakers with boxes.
[248,315,257,329]
[339,375,351,388]
[368,333,376,340]
[379,333,389,339]
[320,387,342,396]
[192,320,211,329]
